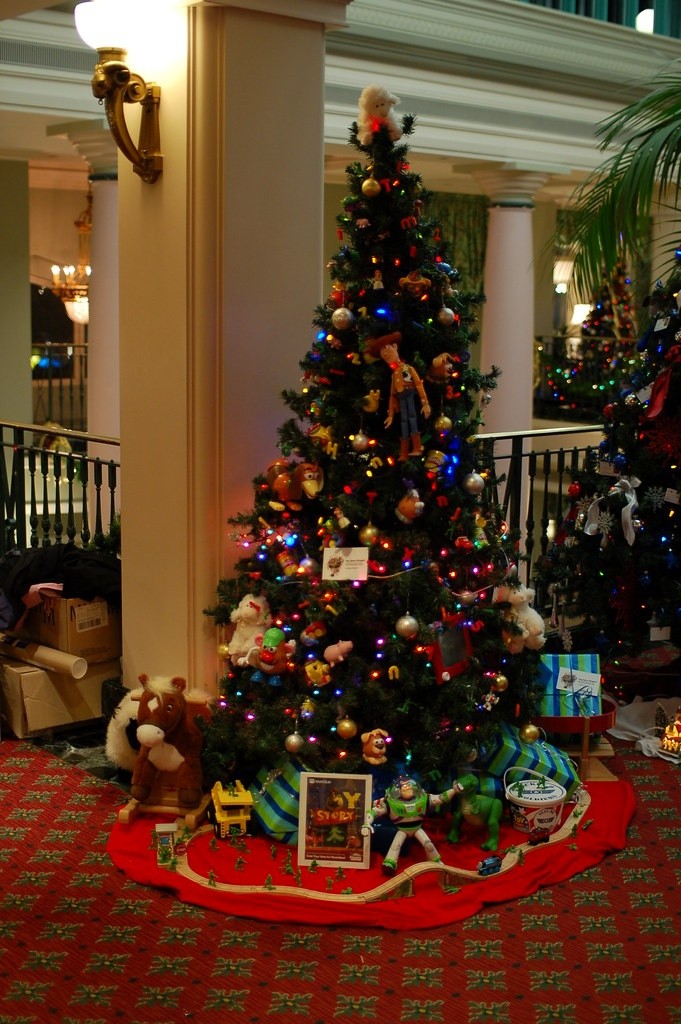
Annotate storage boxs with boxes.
[36,591,120,668]
[0,656,118,743]
[538,652,603,747]
[443,767,505,802]
[481,723,580,804]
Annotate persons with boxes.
[369,332,430,460]
[361,781,458,872]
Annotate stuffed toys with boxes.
[226,594,273,666]
[106,672,218,805]
[493,583,547,654]
[357,83,403,146]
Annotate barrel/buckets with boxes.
[503,766,567,833]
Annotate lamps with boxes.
[49,188,91,324]
[76,1,163,184]
